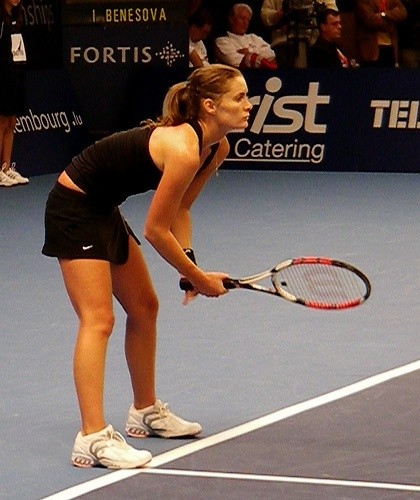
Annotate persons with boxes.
[189,10,212,68]
[354,0,408,69]
[0,0,30,187]
[214,3,278,70]
[261,0,340,50]
[308,0,358,69]
[41,64,253,470]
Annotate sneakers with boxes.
[71,425,152,469]
[4,162,30,184]
[125,399,203,439]
[0,161,19,187]
